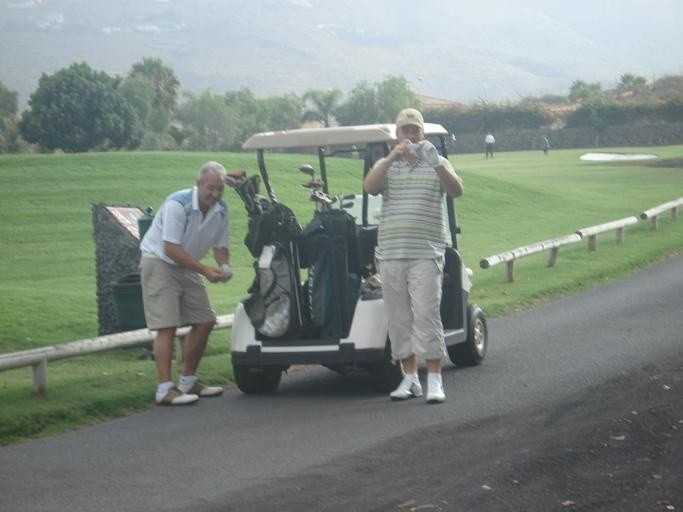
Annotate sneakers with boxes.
[390,378,423,400]
[176,383,223,397]
[154,386,199,404]
[426,373,446,403]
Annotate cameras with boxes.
[407,144,425,154]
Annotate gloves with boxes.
[418,140,440,168]
[220,264,233,282]
[387,138,413,162]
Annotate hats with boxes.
[396,108,425,128]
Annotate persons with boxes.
[364,108,463,402]
[542,136,550,154]
[485,132,495,157]
[140,161,233,405]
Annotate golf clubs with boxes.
[224,171,271,215]
[299,164,355,210]
